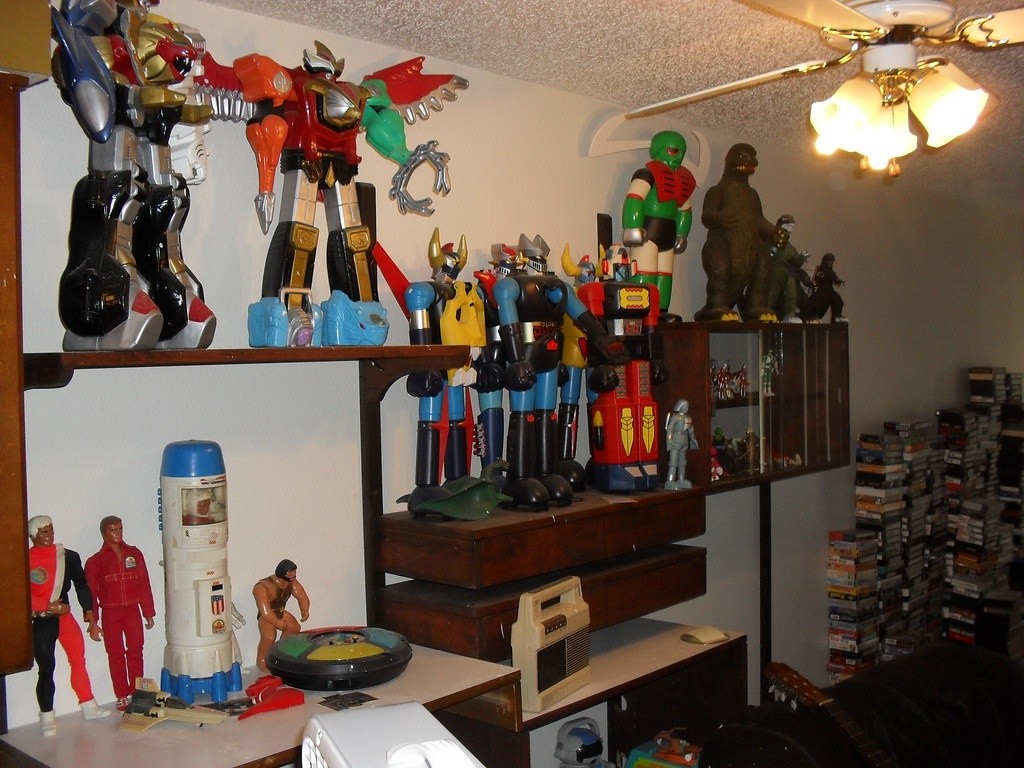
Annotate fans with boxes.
[625,0,1024,121]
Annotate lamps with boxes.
[811,52,1000,172]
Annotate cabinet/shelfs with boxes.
[0,0,854,768]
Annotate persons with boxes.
[203,41,470,347]
[28,516,111,737]
[621,130,696,323]
[84,515,156,714]
[554,717,616,768]
[253,559,310,672]
[372,228,669,521]
[710,348,780,482]
[664,400,699,492]
[51,0,217,350]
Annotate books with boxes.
[826,367,1024,687]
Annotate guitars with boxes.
[761,660,901,768]
